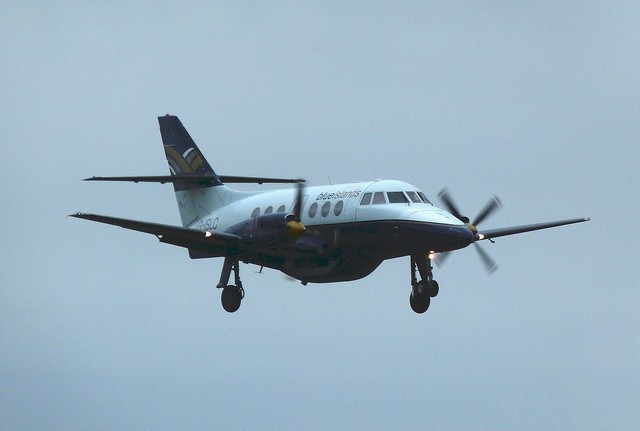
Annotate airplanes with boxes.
[68,114,590,315]
